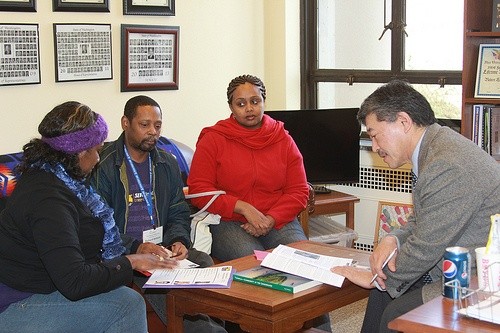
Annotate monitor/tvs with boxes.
[264,108,362,185]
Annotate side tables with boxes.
[310,190,360,249]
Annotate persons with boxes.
[331,81,500,333]
[57,31,110,72]
[15,31,36,42]
[0,100,178,333]
[187,75,330,333]
[0,58,36,77]
[91,95,227,333]
[16,44,37,56]
[130,40,171,76]
[5,46,11,54]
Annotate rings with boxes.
[247,229,251,232]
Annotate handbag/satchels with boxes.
[184,191,227,255]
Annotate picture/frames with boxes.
[0,0,36,13]
[0,23,41,86]
[52,0,110,13]
[373,200,413,251]
[53,23,113,82]
[120,24,180,92]
[123,0,175,16]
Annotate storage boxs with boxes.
[308,217,357,248]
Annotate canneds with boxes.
[442,247,471,300]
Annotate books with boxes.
[138,246,200,274]
[473,104,500,157]
[260,244,354,287]
[143,267,237,289]
[235,267,322,293]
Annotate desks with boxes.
[167,240,373,333]
[387,276,500,333]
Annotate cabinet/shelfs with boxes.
[461,0,500,162]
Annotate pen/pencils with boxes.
[370,247,397,284]
